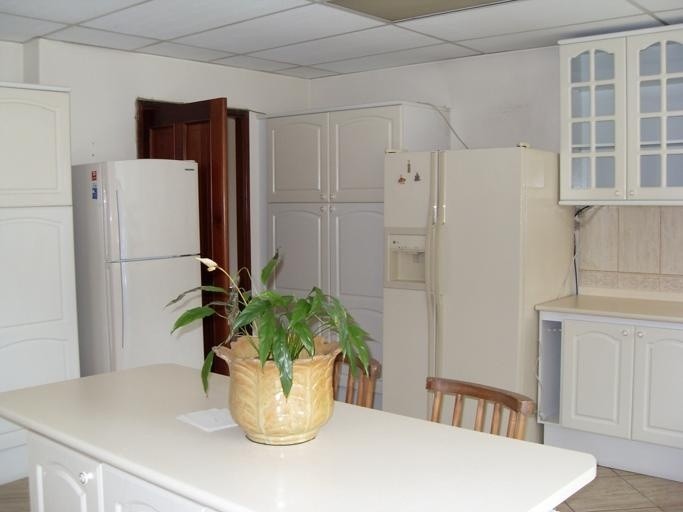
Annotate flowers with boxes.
[163,248,372,403]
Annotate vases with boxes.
[213,337,343,445]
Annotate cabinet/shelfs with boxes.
[0,82,81,488]
[557,23,683,207]
[258,101,450,409]
[559,319,682,450]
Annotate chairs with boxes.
[333,353,381,409]
[425,377,535,441]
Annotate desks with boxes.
[0,363,597,511]
